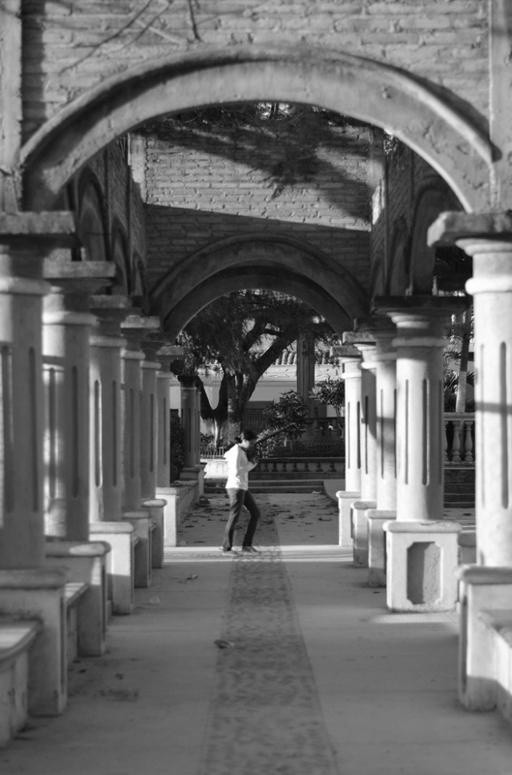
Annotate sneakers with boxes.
[223,544,261,558]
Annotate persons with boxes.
[222,431,261,556]
